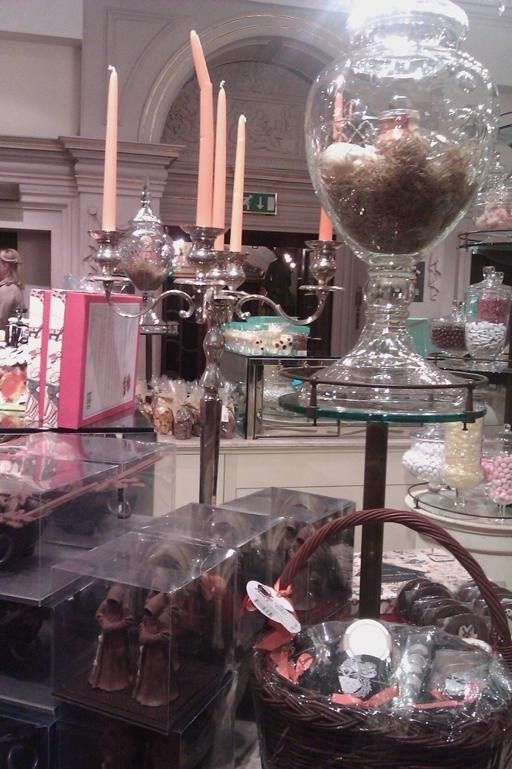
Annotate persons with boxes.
[0,247,24,329]
[263,246,292,312]
[88,494,339,704]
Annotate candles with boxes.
[212,88,227,252]
[101,70,119,233]
[317,92,343,245]
[229,115,247,253]
[189,29,214,228]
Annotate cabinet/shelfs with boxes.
[402,223,512,586]
[273,358,495,615]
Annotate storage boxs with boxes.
[24,289,146,431]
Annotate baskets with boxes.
[250,507,512,768]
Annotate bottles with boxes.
[402,423,446,496]
[473,162,511,232]
[428,298,466,354]
[462,265,510,361]
[443,420,481,507]
[480,425,510,521]
